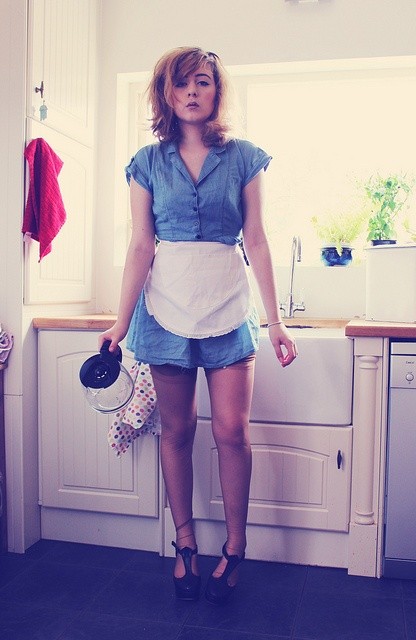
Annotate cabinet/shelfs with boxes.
[164,334,354,575]
[37,330,162,555]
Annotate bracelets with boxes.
[267,321,284,328]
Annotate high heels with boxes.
[171,540,200,600]
[206,540,247,603]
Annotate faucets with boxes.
[285,233,302,317]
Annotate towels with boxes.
[21,138,67,262]
[108,361,161,460]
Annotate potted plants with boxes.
[308,200,381,266]
[364,169,416,246]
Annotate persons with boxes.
[98,47,299,605]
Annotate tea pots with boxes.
[80,341,135,416]
